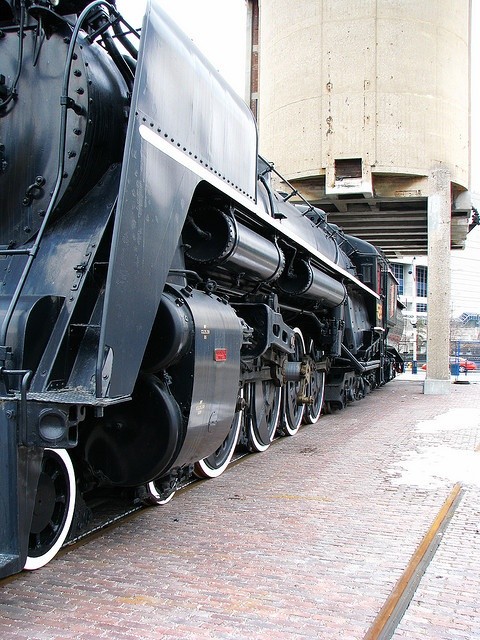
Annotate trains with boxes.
[0,0,405,577]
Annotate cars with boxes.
[422,356,477,373]
